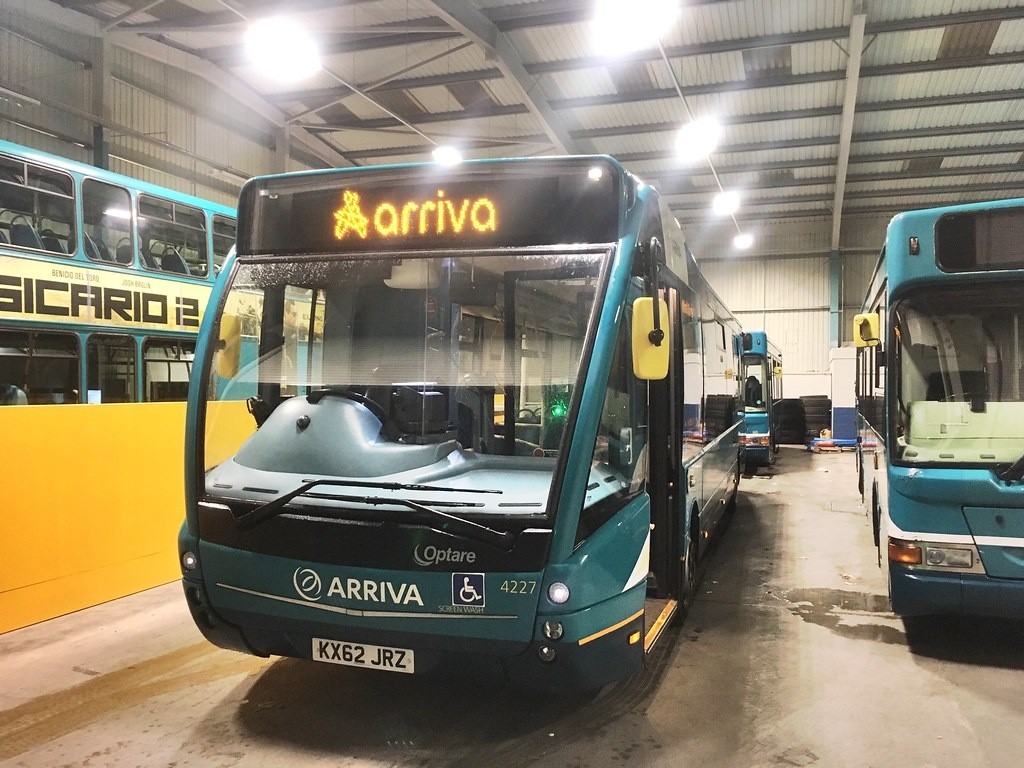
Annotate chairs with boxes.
[0,384,29,406]
[360,345,421,427]
[925,370,987,401]
[0,215,192,276]
[515,405,568,450]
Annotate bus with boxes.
[180,152,746,677]
[853,198,1024,620]
[0,138,325,407]
[744,330,782,465]
